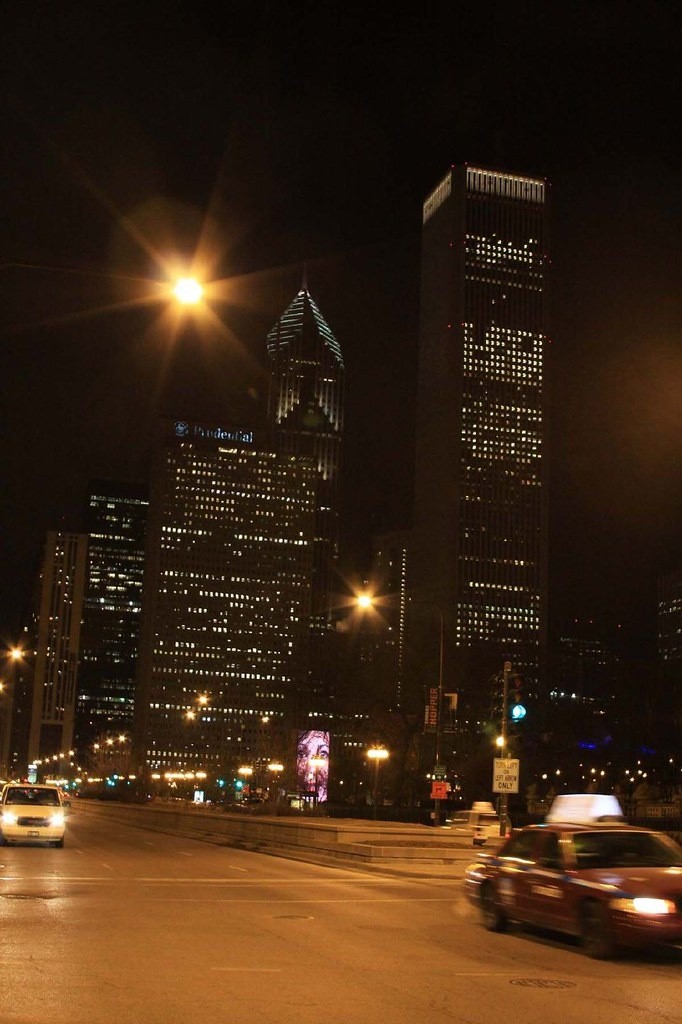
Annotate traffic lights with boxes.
[507,674,526,720]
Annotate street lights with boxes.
[366,748,390,820]
[354,593,444,826]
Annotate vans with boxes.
[448,809,513,845]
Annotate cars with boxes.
[460,821,682,964]
[0,782,71,849]
